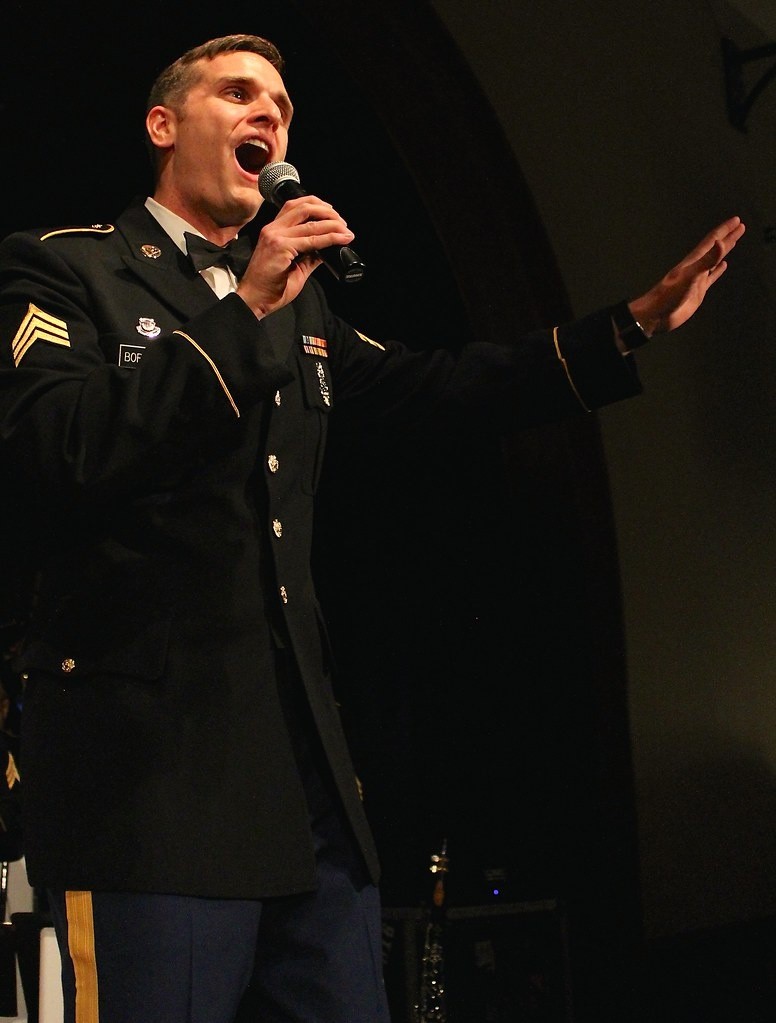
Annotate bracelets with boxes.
[611,298,654,351]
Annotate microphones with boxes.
[258,161,367,284]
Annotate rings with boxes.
[708,269,712,276]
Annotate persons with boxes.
[1,35,746,1020]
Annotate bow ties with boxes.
[184,231,253,276]
